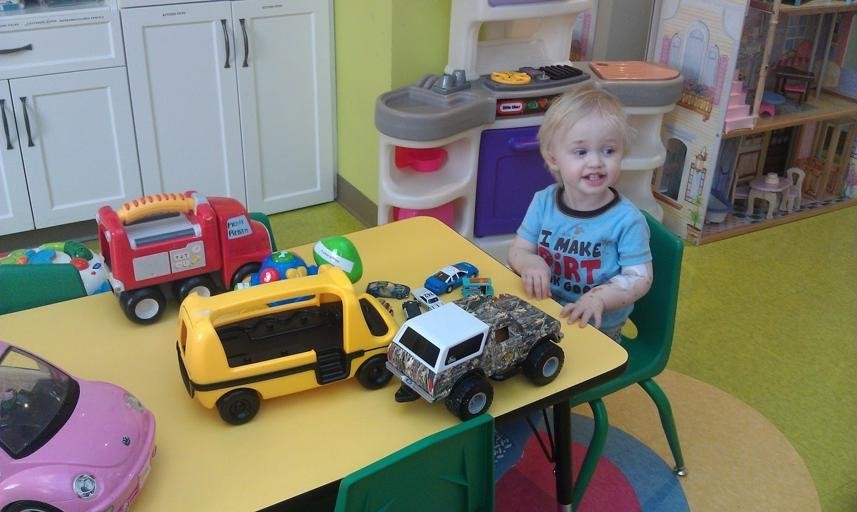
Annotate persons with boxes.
[464,83,654,484]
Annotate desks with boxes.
[0,215,629,512]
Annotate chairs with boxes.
[0,263,88,315]
[335,413,496,512]
[570,208,688,512]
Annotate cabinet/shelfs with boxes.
[0,8,144,236]
[642,0,857,246]
[119,0,337,216]
